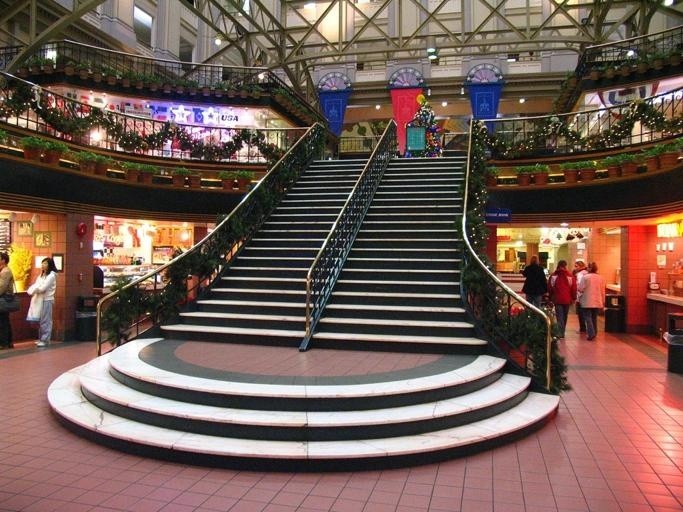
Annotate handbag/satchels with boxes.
[0,293,20,313]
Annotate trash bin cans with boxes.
[603,294,625,331]
[74,293,100,342]
[665,312,683,374]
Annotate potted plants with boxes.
[483,142,680,187]
[20,136,255,192]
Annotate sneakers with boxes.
[587,335,595,341]
[36,341,49,347]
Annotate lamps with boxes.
[424,36,439,60]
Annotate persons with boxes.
[520,255,548,309]
[33,258,58,348]
[0,253,15,351]
[577,261,606,340]
[548,260,578,339]
[573,261,588,334]
